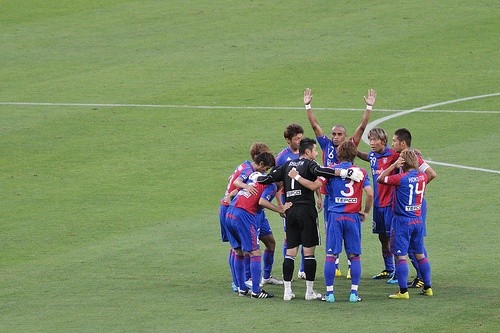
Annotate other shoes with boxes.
[335,269,341,276]
[347,269,351,279]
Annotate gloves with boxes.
[340,167,364,183]
[250,172,261,183]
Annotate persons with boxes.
[356,128,403,284]
[220,142,293,299]
[275,124,322,279]
[248,137,364,300]
[288,140,373,303]
[377,148,434,299]
[304,88,377,279]
[387,127,436,288]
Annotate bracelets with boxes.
[305,104,311,110]
[366,105,372,111]
[295,174,301,180]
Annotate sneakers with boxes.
[238,289,252,297]
[420,288,433,295]
[251,289,274,299]
[261,274,283,285]
[407,278,423,288]
[321,293,335,302]
[305,293,322,300]
[245,280,263,288]
[349,293,362,302]
[389,290,409,299]
[373,271,393,279]
[284,294,295,300]
[386,277,398,284]
[298,271,305,279]
